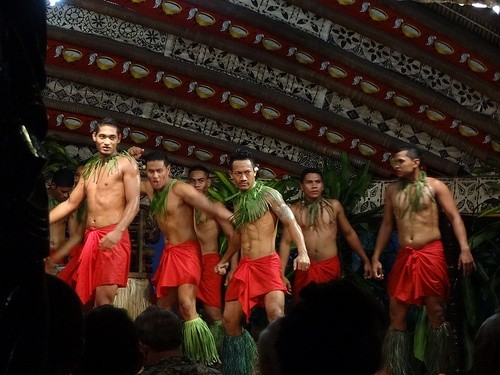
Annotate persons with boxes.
[276,167,372,302]
[53,161,84,296]
[467,310,500,375]
[371,146,475,375]
[48,118,141,308]
[214,150,310,375]
[2,273,86,375]
[185,166,239,361]
[125,145,235,366]
[257,317,290,375]
[83,303,146,374]
[274,277,389,375]
[45,167,80,277]
[134,307,223,375]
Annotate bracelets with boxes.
[227,214,236,223]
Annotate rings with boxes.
[305,267,308,269]
